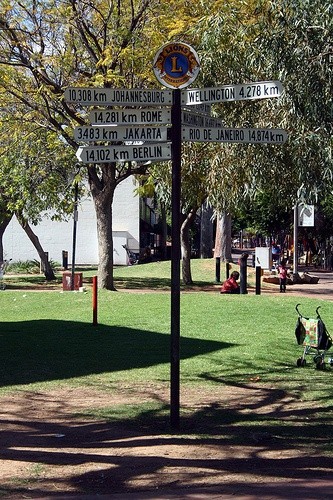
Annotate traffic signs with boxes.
[64,86,174,162]
[181,80,289,145]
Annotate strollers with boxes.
[295,303,333,370]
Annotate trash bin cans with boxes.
[63,273,82,292]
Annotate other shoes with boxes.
[280,291,282,292]
[283,291,285,292]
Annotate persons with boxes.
[277,261,290,293]
[220,271,248,294]
